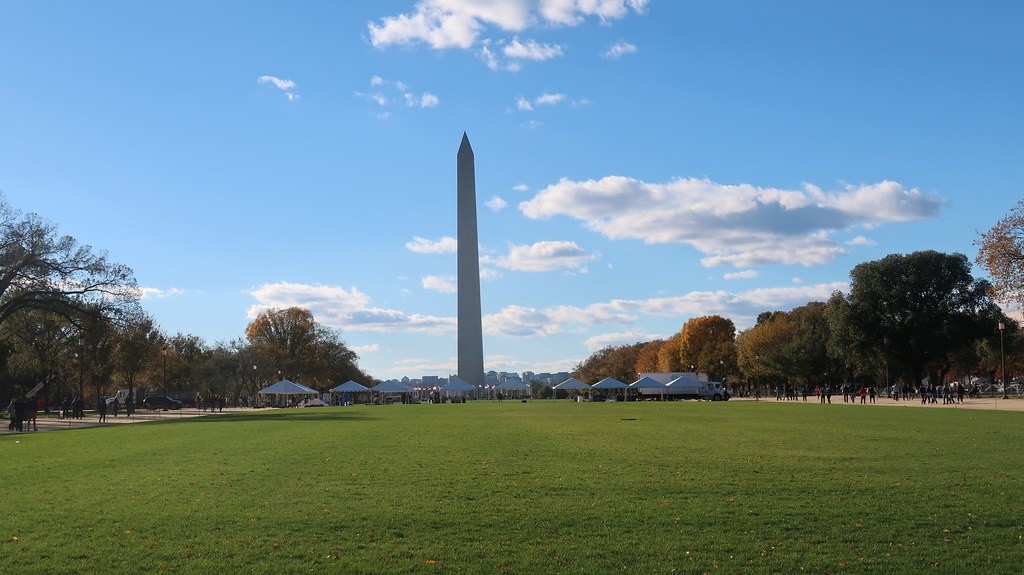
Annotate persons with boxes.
[868,387,876,404]
[815,386,831,404]
[343,392,350,406]
[731,388,735,396]
[755,389,760,401]
[776,385,807,401]
[919,382,980,404]
[195,394,224,412]
[7,394,134,433]
[842,383,856,403]
[744,390,750,397]
[723,388,727,401]
[892,383,915,401]
[496,391,503,403]
[860,387,867,404]
[401,392,412,404]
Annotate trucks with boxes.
[105,387,178,410]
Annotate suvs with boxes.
[143,396,183,410]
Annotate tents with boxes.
[258,380,320,407]
[629,377,665,399]
[666,375,703,399]
[370,379,410,404]
[592,377,629,400]
[553,377,592,401]
[329,380,373,405]
[439,379,475,403]
[496,378,527,398]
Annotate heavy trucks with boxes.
[638,371,733,401]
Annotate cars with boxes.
[979,383,1023,394]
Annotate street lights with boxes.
[720,359,723,380]
[883,337,891,398]
[999,320,1007,399]
[252,363,256,406]
[162,348,167,411]
[78,336,85,418]
